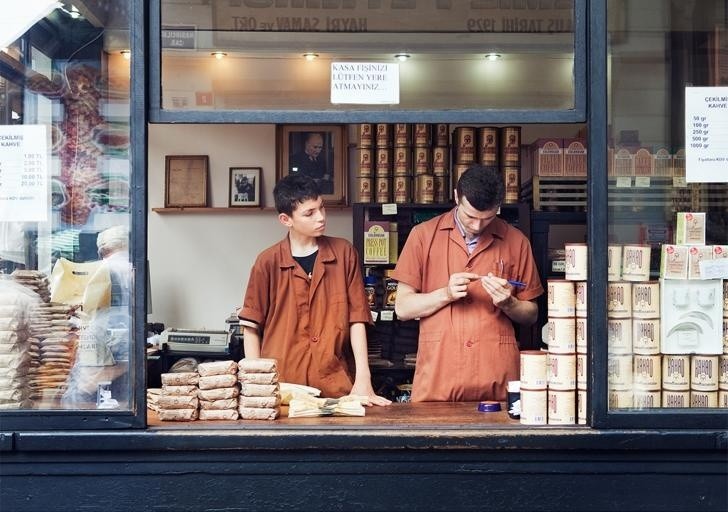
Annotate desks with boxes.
[145,329,244,388]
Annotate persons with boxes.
[292,133,333,194]
[392,165,544,404]
[237,174,395,408]
[88,224,134,406]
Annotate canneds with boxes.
[519,241,728,426]
[356,122,522,203]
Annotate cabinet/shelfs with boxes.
[352,202,532,402]
[524,177,728,351]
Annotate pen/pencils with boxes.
[479,276,527,286]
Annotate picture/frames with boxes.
[165,155,208,207]
[229,166,262,207]
[276,124,348,208]
